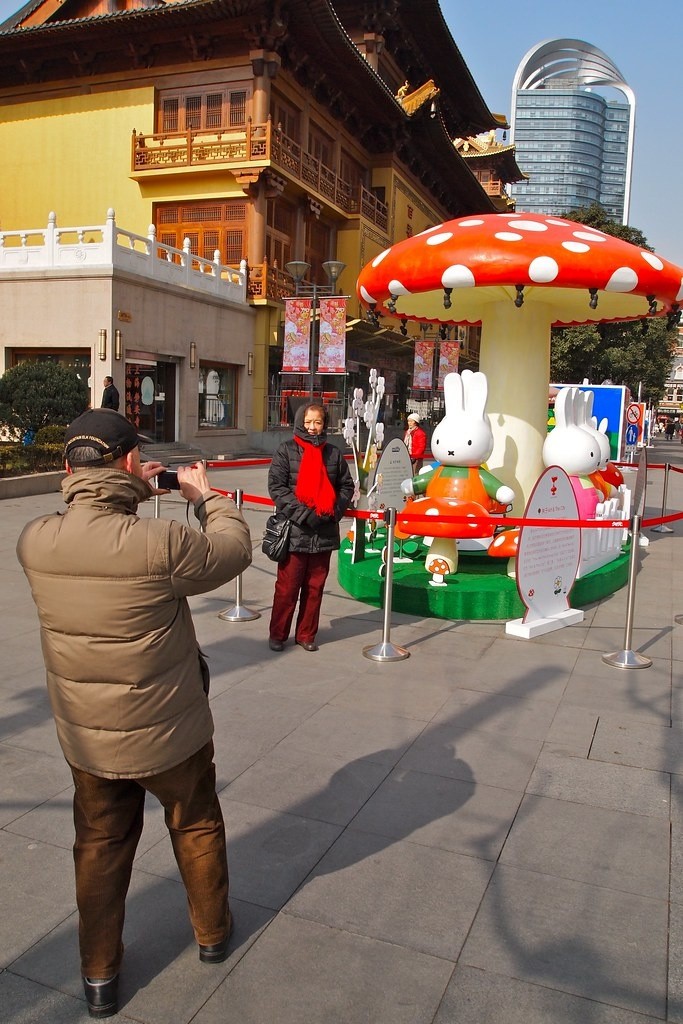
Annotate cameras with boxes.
[157,471,181,490]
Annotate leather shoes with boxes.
[83,975,119,1019]
[199,911,233,964]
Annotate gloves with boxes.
[307,512,323,529]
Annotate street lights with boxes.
[280,260,347,405]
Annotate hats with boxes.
[407,412,420,423]
[64,408,156,468]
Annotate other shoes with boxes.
[295,637,317,651]
[269,637,283,651]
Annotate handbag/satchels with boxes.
[262,515,293,561]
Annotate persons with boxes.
[15,409,253,1018]
[268,401,354,652]
[403,413,426,501]
[658,417,683,445]
[100,376,120,412]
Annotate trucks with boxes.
[547,378,630,466]
